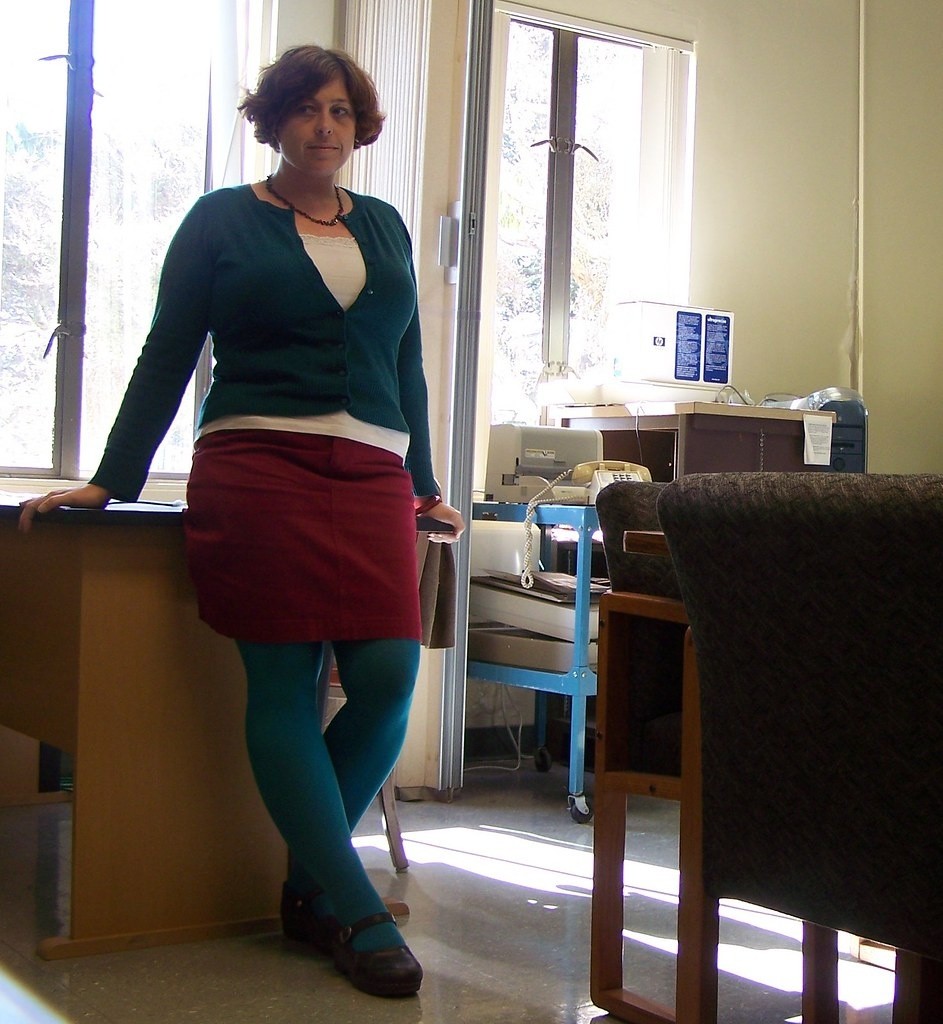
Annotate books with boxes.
[533,572,607,595]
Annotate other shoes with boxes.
[281,881,332,954]
[320,912,423,994]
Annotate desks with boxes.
[0,493,412,966]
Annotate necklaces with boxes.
[266,174,343,226]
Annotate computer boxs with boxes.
[818,400,867,475]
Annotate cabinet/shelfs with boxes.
[555,404,837,779]
[470,502,602,830]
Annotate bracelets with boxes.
[414,493,443,516]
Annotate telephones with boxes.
[569,459,652,504]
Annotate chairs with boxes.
[325,541,457,874]
[594,472,943,1024]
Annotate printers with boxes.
[484,424,604,502]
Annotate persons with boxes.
[20,47,465,995]
